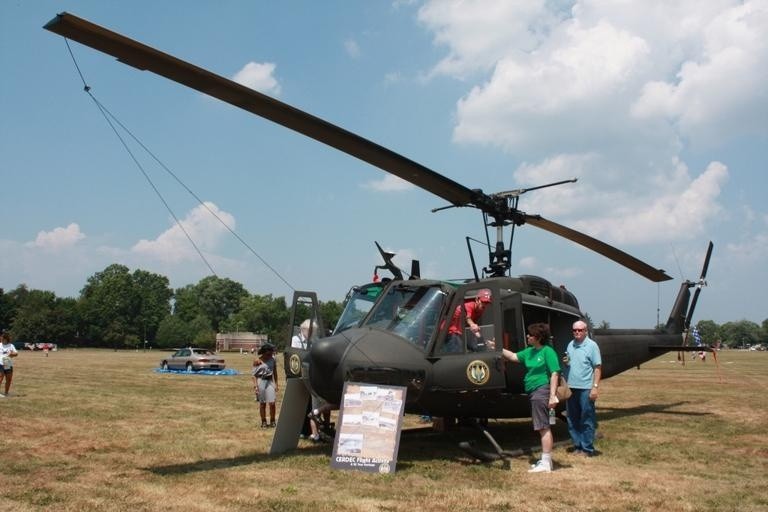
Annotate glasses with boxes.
[573,329,582,331]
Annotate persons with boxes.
[0,332,19,394]
[562,320,604,457]
[483,322,562,473]
[43,343,49,357]
[290,317,321,442]
[440,287,494,352]
[252,344,278,428]
[309,318,336,437]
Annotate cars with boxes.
[158,347,226,372]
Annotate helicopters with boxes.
[37,8,719,479]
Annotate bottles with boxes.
[549,408,556,424]
[254,386,261,402]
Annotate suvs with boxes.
[12,341,30,350]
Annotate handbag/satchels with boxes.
[556,376,572,401]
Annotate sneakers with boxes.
[529,460,554,472]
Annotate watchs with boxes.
[591,383,599,388]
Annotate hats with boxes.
[479,288,492,303]
